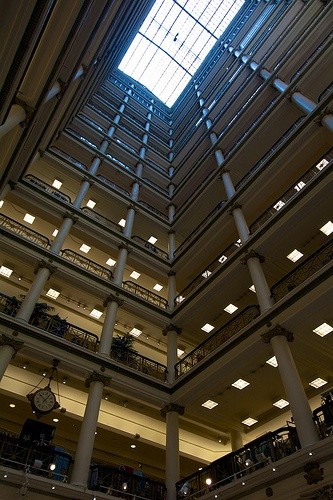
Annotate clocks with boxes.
[28,382,62,418]
